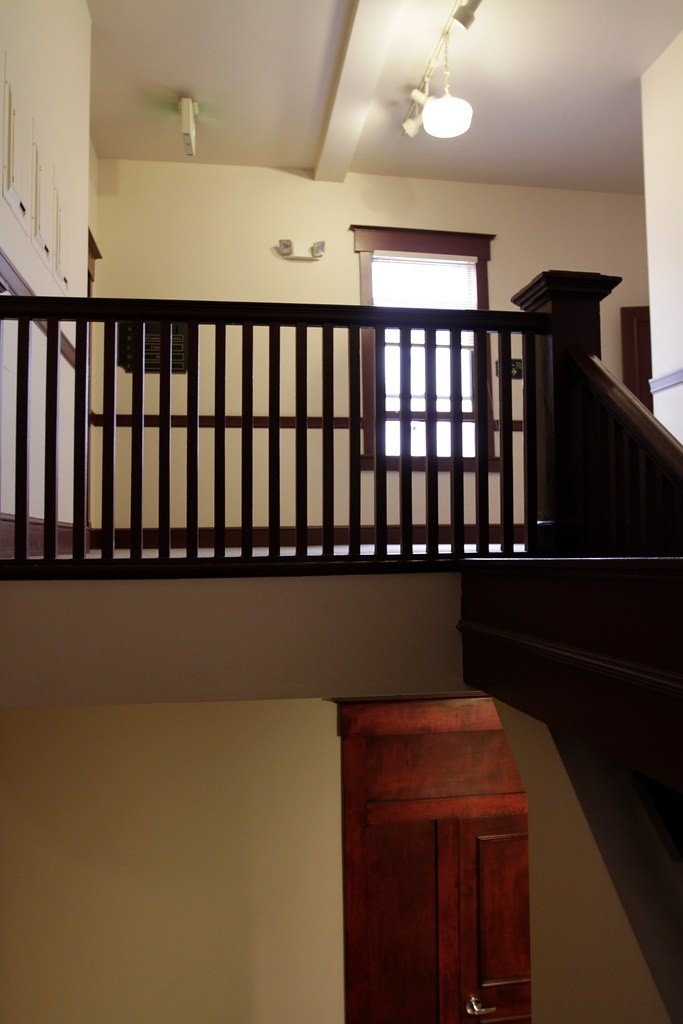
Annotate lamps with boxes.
[279,239,325,260]
[402,32,473,139]
[453,0,481,28]
